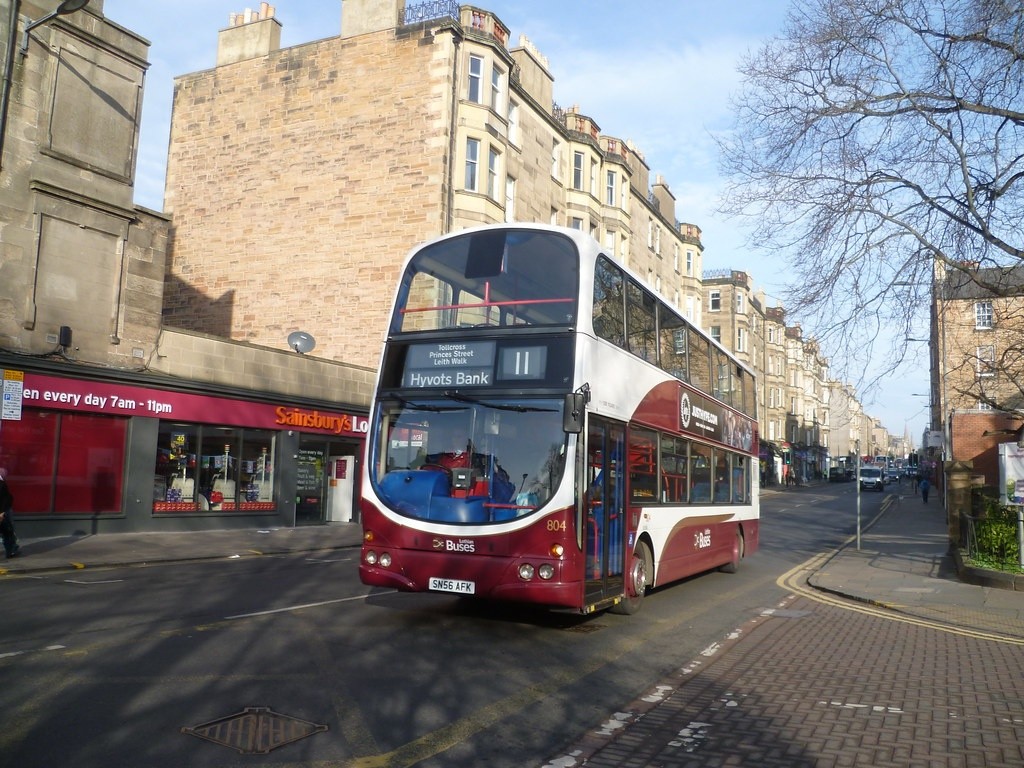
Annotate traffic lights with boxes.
[786,453,791,465]
[908,454,912,466]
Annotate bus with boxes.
[357,224,760,616]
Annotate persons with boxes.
[785,468,801,487]
[911,475,921,495]
[920,478,930,503]
[823,468,828,480]
[0,468,22,559]
[417,423,472,470]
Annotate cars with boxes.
[874,456,917,485]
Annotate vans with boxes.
[859,467,884,493]
